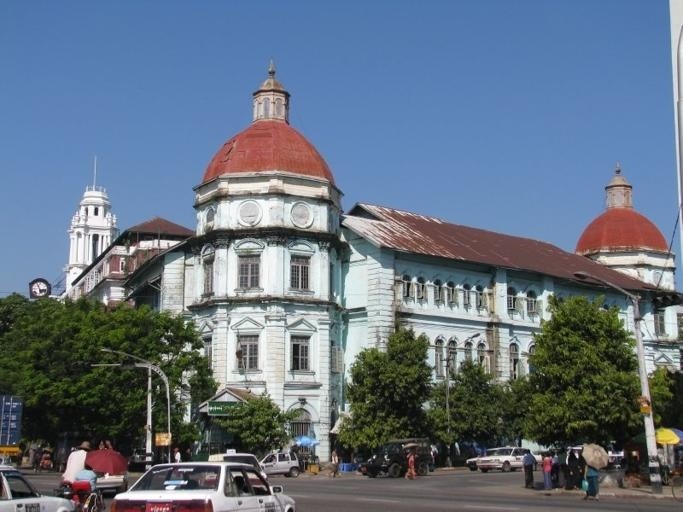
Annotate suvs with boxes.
[357,435,434,477]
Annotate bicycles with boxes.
[670,476,683,503]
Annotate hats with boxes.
[78,441,91,449]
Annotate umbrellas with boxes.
[631,425,682,485]
[85,448,129,475]
[293,435,320,472]
[580,443,609,471]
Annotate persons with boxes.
[404,452,417,480]
[172,447,182,462]
[60,439,92,484]
[28,442,41,473]
[270,455,276,462]
[330,445,339,477]
[233,475,250,497]
[582,464,601,500]
[520,448,584,489]
[74,464,98,492]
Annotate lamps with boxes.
[299,398,307,405]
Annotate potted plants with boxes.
[307,452,320,473]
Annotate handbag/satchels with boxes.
[582,480,589,491]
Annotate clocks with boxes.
[29,278,50,299]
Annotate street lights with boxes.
[571,269,665,495]
[446,331,482,435]
[100,347,173,464]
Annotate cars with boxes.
[462,442,638,474]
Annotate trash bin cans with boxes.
[307,463,319,474]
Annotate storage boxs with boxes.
[339,463,358,472]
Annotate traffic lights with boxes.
[121,358,135,368]
